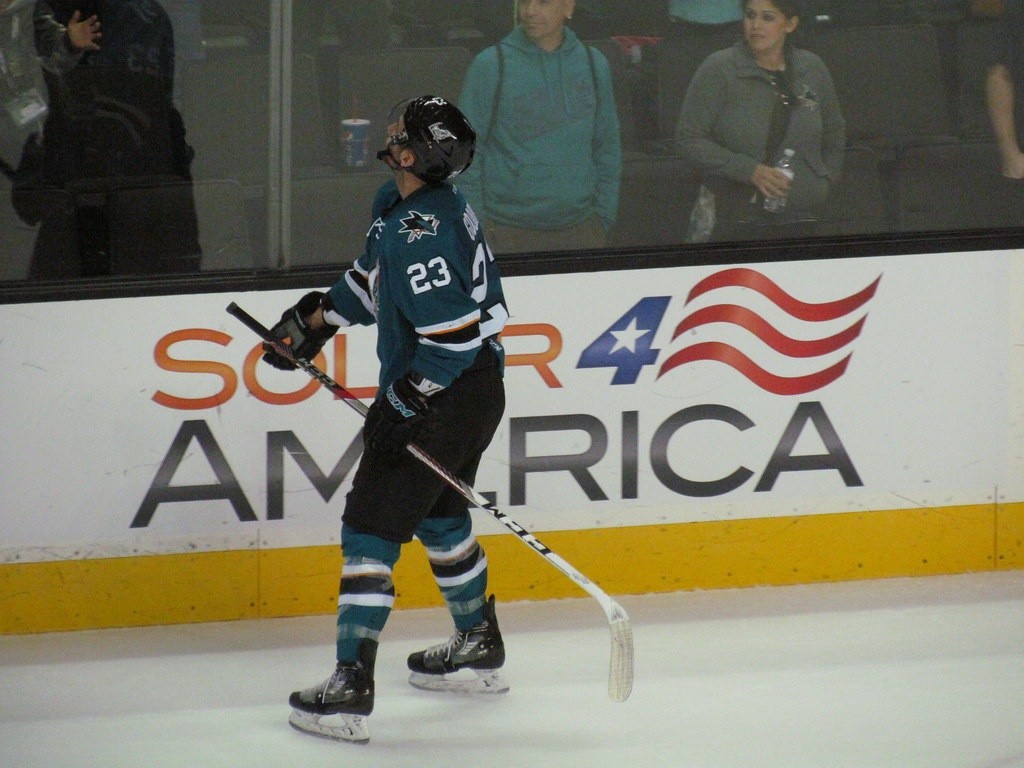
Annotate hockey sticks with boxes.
[224,301,636,704]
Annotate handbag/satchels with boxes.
[680,185,738,244]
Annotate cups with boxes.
[340,119,371,168]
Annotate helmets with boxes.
[392,94,477,183]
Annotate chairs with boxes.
[0,0,1024,282]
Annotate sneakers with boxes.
[407,593,512,696]
[289,639,379,744]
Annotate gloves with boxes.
[362,375,429,454]
[260,291,339,372]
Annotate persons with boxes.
[676,0,848,246]
[668,0,744,91]
[260,97,510,744]
[986,12,1024,179]
[453,1,622,255]
[23,1,201,285]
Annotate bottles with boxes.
[763,148,795,214]
